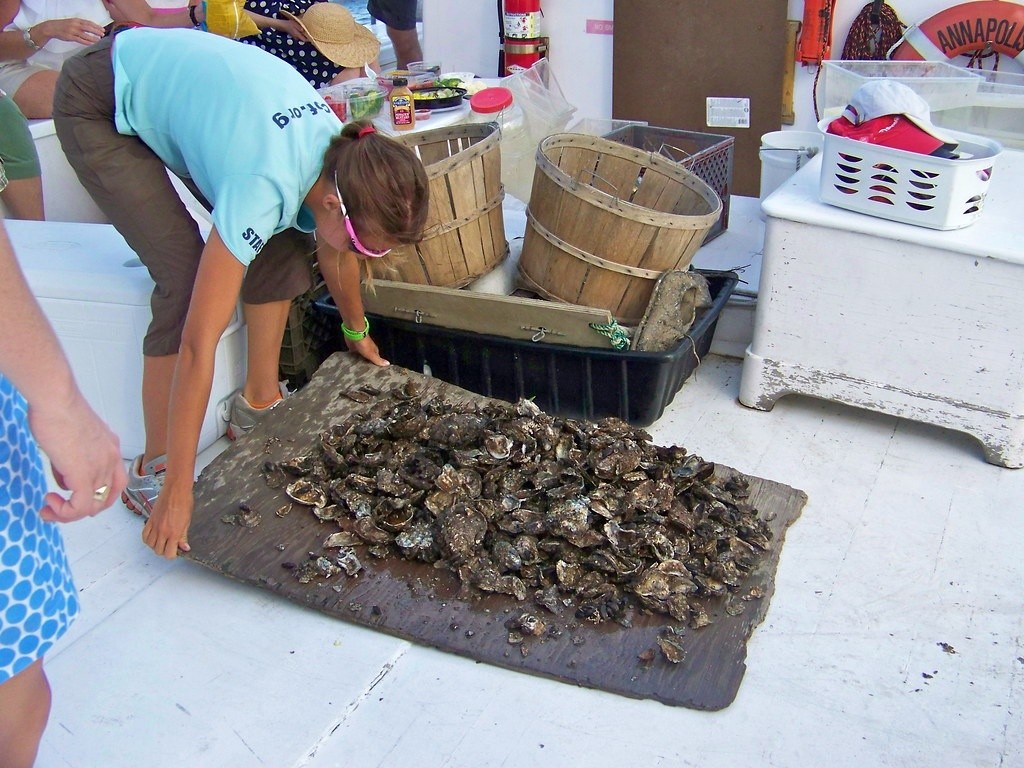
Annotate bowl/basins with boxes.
[316,83,389,124]
[406,61,441,76]
[410,88,468,109]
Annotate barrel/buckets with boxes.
[360,107,504,289]
[517,132,724,326]
[758,131,825,197]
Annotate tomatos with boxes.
[322,96,346,123]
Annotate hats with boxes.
[824,80,988,214]
[279,3,381,67]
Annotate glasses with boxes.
[334,170,392,257]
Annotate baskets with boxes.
[816,117,1003,230]
[604,125,734,247]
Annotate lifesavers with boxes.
[885,1,1024,66]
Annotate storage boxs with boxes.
[821,61,1024,135]
[816,125,1004,229]
[604,124,733,246]
[303,264,740,426]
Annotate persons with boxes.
[0,89,45,221]
[52,27,431,560]
[0,222,130,768]
[0,0,422,119]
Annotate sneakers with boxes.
[121,453,167,522]
[227,380,298,440]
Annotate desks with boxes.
[740,149,1024,468]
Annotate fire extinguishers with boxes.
[501,0,543,78]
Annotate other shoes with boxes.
[629,269,695,353]
[689,271,713,308]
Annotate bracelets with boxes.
[23,27,41,51]
[189,5,201,27]
[340,315,370,341]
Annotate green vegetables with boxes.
[433,78,464,87]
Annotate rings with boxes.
[93,483,110,500]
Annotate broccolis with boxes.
[350,90,384,119]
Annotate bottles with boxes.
[389,78,415,130]
[465,87,536,181]
